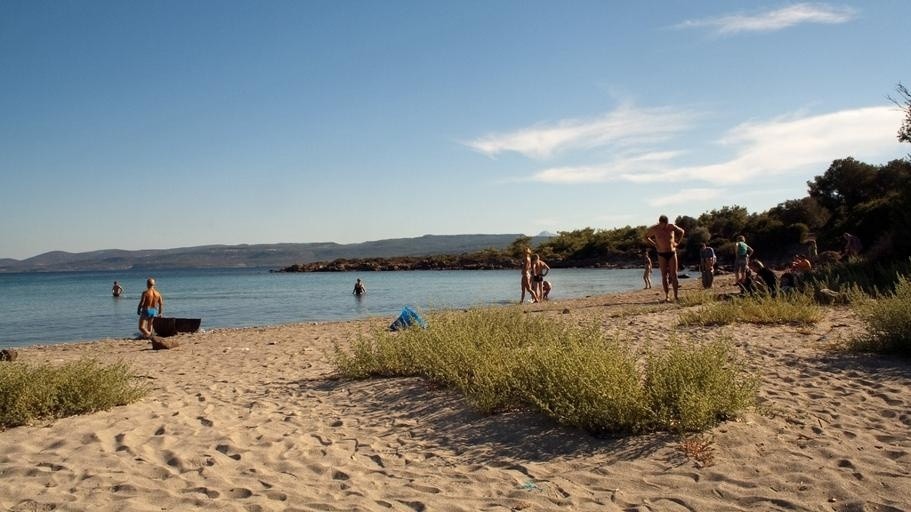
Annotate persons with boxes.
[531,254,550,303]
[136,278,163,340]
[518,247,539,304]
[642,215,862,302]
[112,280,123,297]
[351,278,366,296]
[529,270,537,300]
[543,279,552,299]
